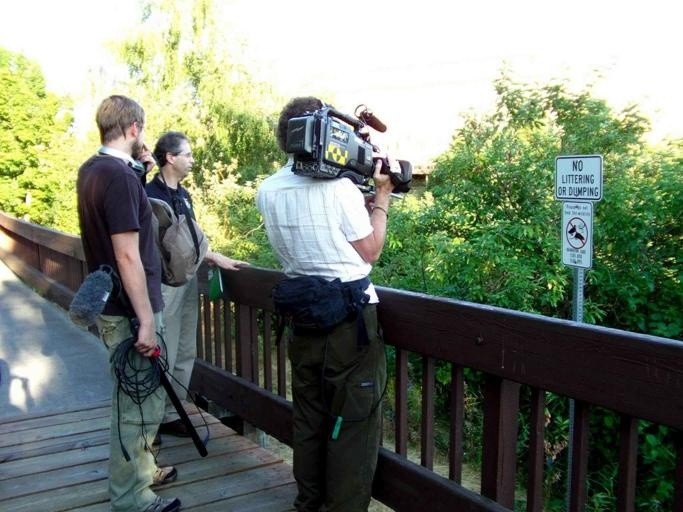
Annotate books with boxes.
[205,261,224,302]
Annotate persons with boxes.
[74,93,182,512]
[141,131,252,448]
[252,96,402,511]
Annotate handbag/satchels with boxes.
[272,273,371,335]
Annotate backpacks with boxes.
[146,193,211,288]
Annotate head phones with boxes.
[96,145,147,179]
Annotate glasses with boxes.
[172,151,193,158]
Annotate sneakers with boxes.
[140,466,178,488]
[157,414,194,438]
[111,493,181,512]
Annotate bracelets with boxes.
[370,204,389,220]
